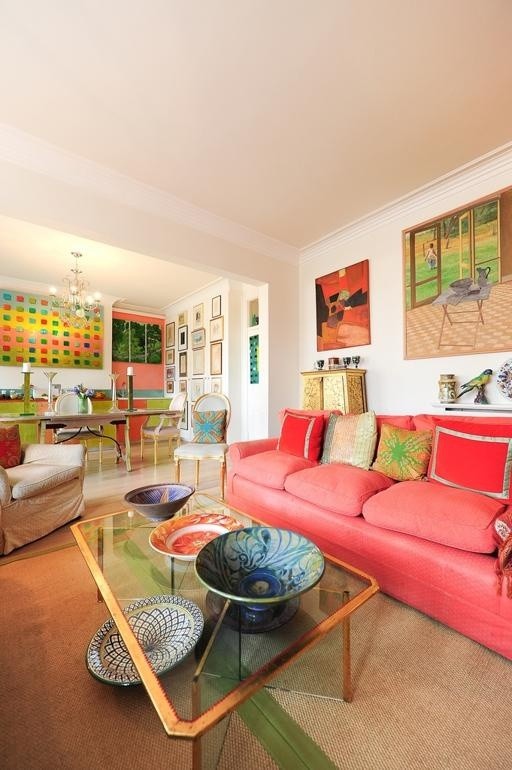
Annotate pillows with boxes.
[426,416,512,506]
[0,424,22,469]
[190,409,226,443]
[276,409,325,466]
[369,422,433,482]
[320,410,376,472]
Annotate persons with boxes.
[424,243,437,268]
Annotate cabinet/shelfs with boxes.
[0,398,173,453]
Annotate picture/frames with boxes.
[164,293,223,431]
[401,185,512,362]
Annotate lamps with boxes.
[49,251,102,329]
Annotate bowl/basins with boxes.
[124,483,193,521]
[195,525,326,611]
[206,590,302,633]
[449,278,472,293]
[17,393,24,400]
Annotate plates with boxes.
[147,558,200,589]
[85,594,204,688]
[151,514,241,560]
[496,357,512,401]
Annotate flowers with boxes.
[73,383,94,399]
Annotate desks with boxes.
[0,407,182,473]
[433,402,511,417]
[433,282,492,350]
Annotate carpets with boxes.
[0,510,510,770]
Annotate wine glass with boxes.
[317,361,324,370]
[343,356,360,370]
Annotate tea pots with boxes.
[477,266,491,287]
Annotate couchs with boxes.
[225,408,512,663]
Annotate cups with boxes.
[10,390,15,400]
[328,358,339,371]
[1,390,6,399]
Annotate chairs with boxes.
[0,442,89,555]
[139,391,188,466]
[173,392,231,500]
[53,392,104,464]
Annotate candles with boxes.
[127,366,134,375]
[22,363,31,371]
